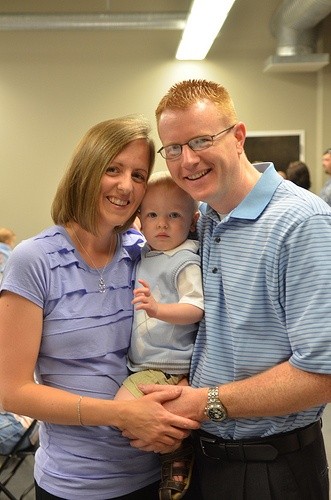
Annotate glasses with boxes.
[157,124,236,159]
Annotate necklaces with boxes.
[68,222,114,293]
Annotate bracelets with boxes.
[77,396,84,424]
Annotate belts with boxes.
[186,422,321,462]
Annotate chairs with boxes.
[0,418,38,500]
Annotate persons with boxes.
[122,79,331,500]
[320,148,331,207]
[125,174,204,500]
[278,161,311,190]
[0,228,16,268]
[0,116,201,500]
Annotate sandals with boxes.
[159,441,194,500]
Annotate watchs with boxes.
[205,385,233,422]
